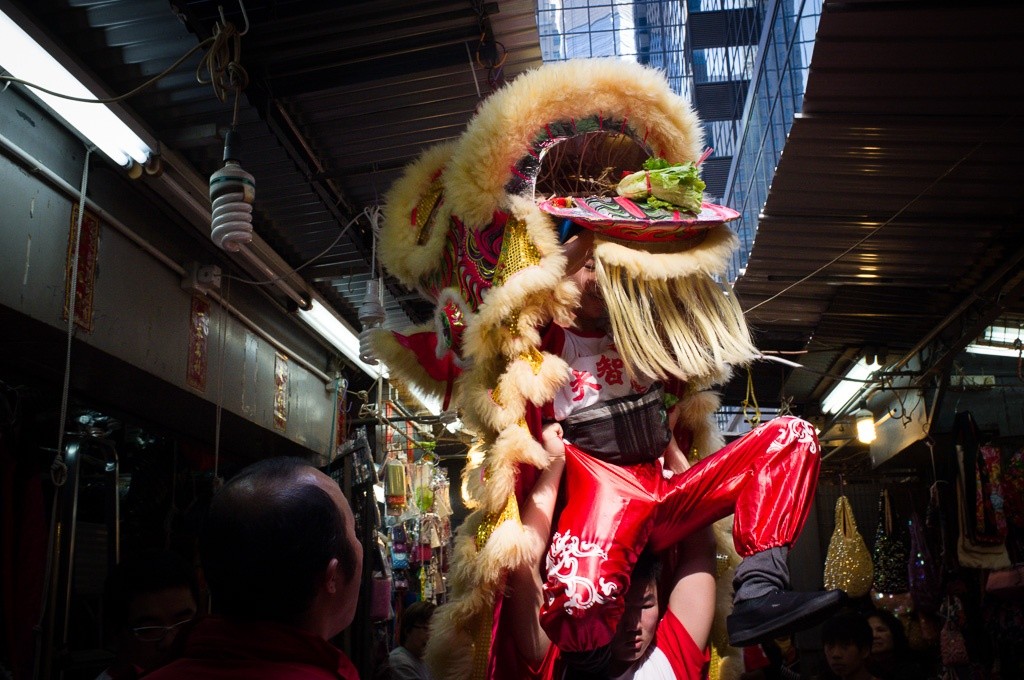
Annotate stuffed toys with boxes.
[368,56,759,680]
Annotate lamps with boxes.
[821,345,887,417]
[0,6,464,437]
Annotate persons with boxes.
[138,455,363,680]
[378,601,436,680]
[504,255,1024,680]
[97,548,201,680]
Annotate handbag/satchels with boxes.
[874,490,910,594]
[909,511,936,590]
[958,536,1011,568]
[824,494,874,596]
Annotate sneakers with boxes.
[726,589,846,647]
